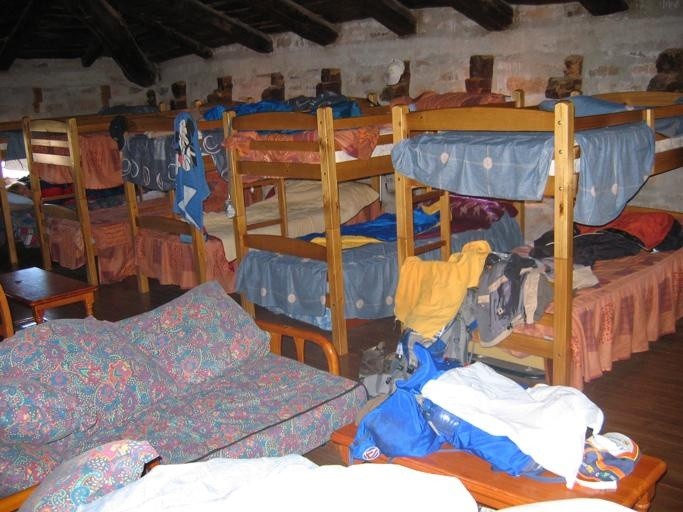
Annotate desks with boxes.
[331,384,668,512]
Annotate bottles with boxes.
[423,399,459,433]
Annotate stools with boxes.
[1,266,100,324]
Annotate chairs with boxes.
[1,293,364,512]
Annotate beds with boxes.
[2,78,680,384]
